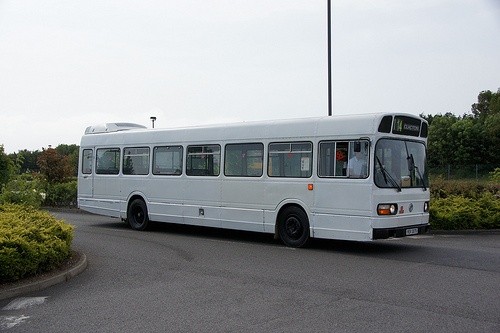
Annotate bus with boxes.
[77,112,433,249]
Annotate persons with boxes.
[346,151,366,178]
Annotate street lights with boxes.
[150,117,156,129]
